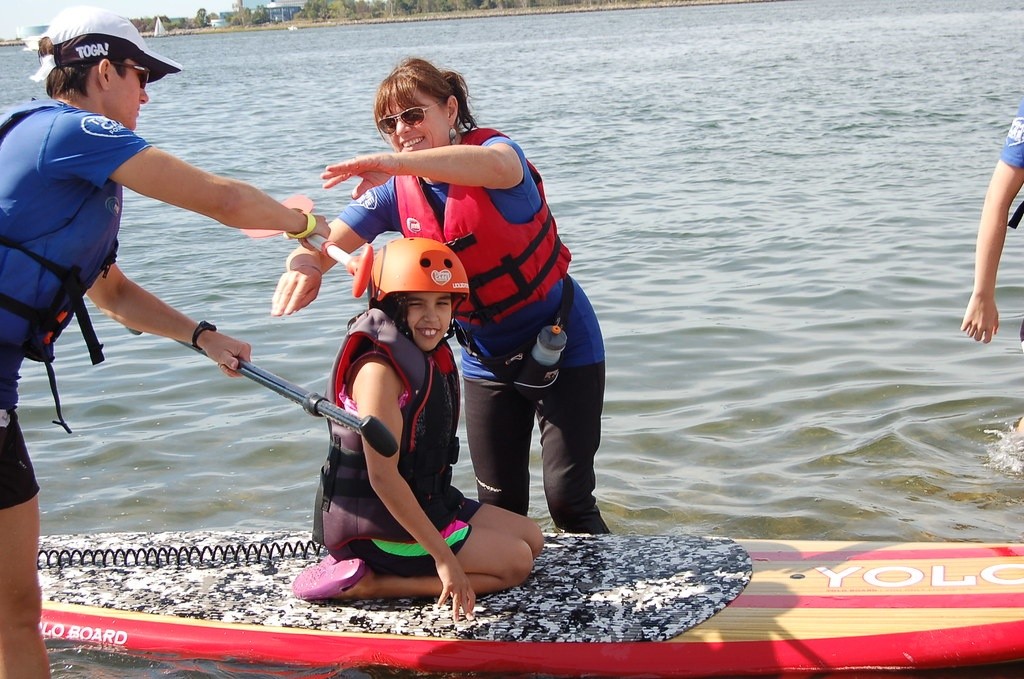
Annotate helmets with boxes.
[371,238,469,303]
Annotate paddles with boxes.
[246,196,375,298]
[129,328,399,458]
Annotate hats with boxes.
[29,6,182,83]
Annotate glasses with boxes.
[378,101,445,135]
[96,61,150,89]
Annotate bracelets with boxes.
[284,209,316,240]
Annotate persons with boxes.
[959,90,1024,432]
[292,237,546,626]
[272,56,611,534]
[0,5,330,678]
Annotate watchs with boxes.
[190,320,217,351]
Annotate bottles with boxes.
[532,325,567,367]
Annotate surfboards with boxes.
[36,532,1024,675]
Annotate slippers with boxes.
[320,554,339,565]
[292,559,365,601]
